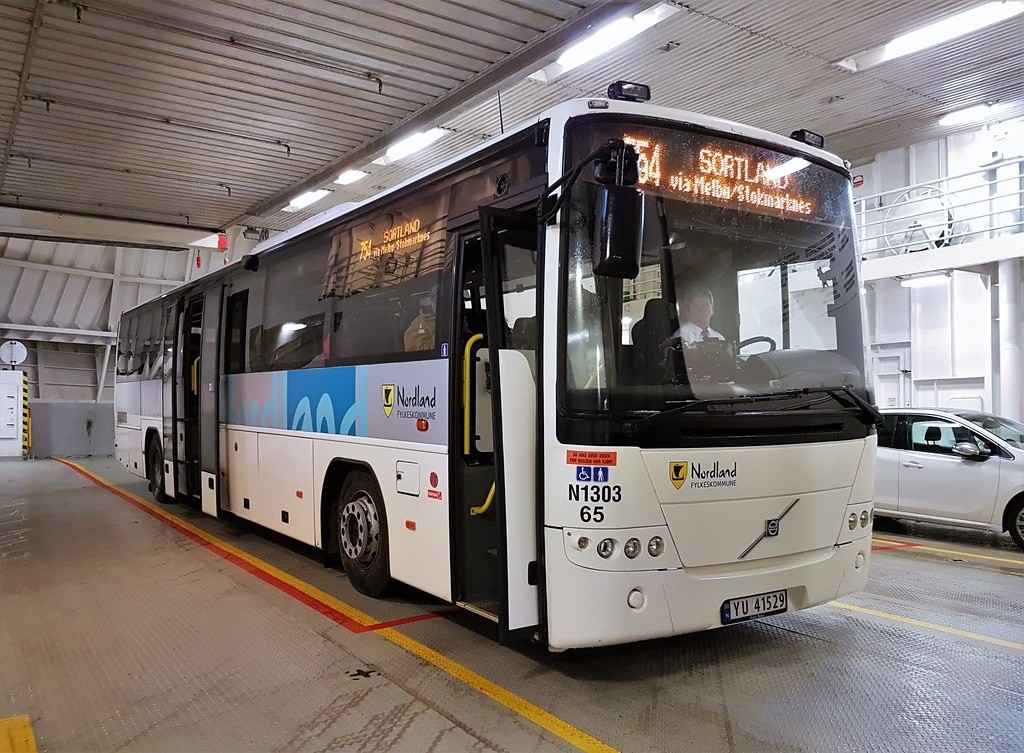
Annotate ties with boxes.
[701,330,709,338]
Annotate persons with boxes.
[673,286,726,349]
[403,284,438,352]
[302,323,368,368]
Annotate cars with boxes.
[873,409,1023,553]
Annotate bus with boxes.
[114,80,886,657]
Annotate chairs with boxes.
[922,426,943,453]
[632,298,673,356]
